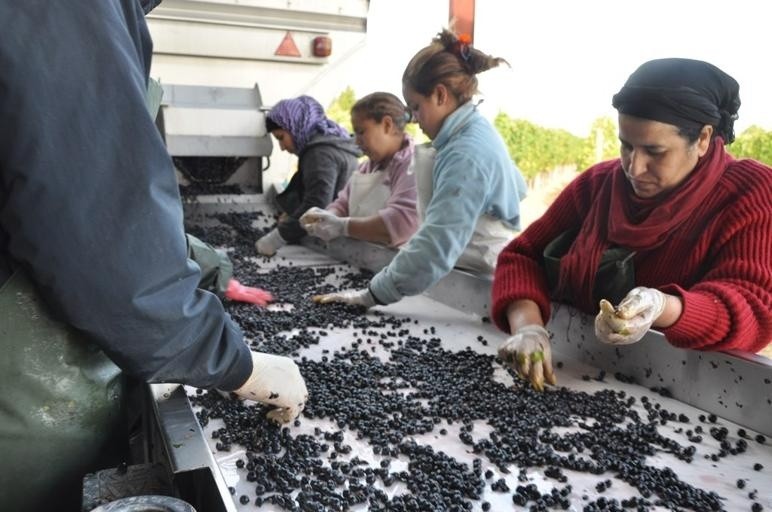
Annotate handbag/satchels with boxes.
[543,225,637,312]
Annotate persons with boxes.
[254,95,366,257]
[299,92,424,249]
[314,26,528,309]
[0,0,309,512]
[490,58,772,393]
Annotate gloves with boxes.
[232,351,308,421]
[225,278,274,307]
